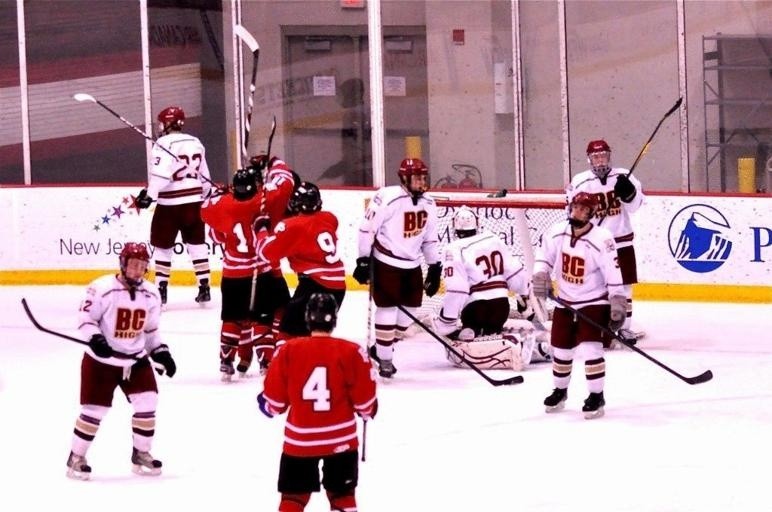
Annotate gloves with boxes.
[609,295,627,332]
[253,213,271,233]
[150,344,175,377]
[89,334,112,357]
[424,261,441,297]
[135,189,152,208]
[518,295,534,321]
[353,256,374,284]
[614,175,636,202]
[533,272,553,297]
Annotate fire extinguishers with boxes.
[433,164,483,190]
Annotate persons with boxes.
[565,140,643,344]
[237,166,291,372]
[354,159,443,377]
[258,293,377,512]
[531,192,627,411]
[437,209,530,341]
[67,242,175,472]
[250,181,346,347]
[137,108,211,303]
[202,156,294,375]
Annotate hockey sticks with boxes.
[547,290,713,384]
[233,24,260,171]
[595,97,683,226]
[248,116,274,322]
[74,92,225,194]
[372,280,523,386]
[21,298,166,372]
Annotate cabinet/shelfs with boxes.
[701,30,771,193]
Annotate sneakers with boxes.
[195,286,210,302]
[68,452,91,472]
[221,358,271,374]
[132,447,162,468]
[618,330,637,344]
[583,391,604,411]
[370,346,397,377]
[544,389,567,406]
[157,288,167,304]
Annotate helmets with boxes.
[120,243,149,274]
[233,166,262,198]
[398,158,429,187]
[305,292,339,322]
[292,181,321,212]
[453,207,478,231]
[571,193,598,217]
[157,107,185,132]
[587,140,610,174]
